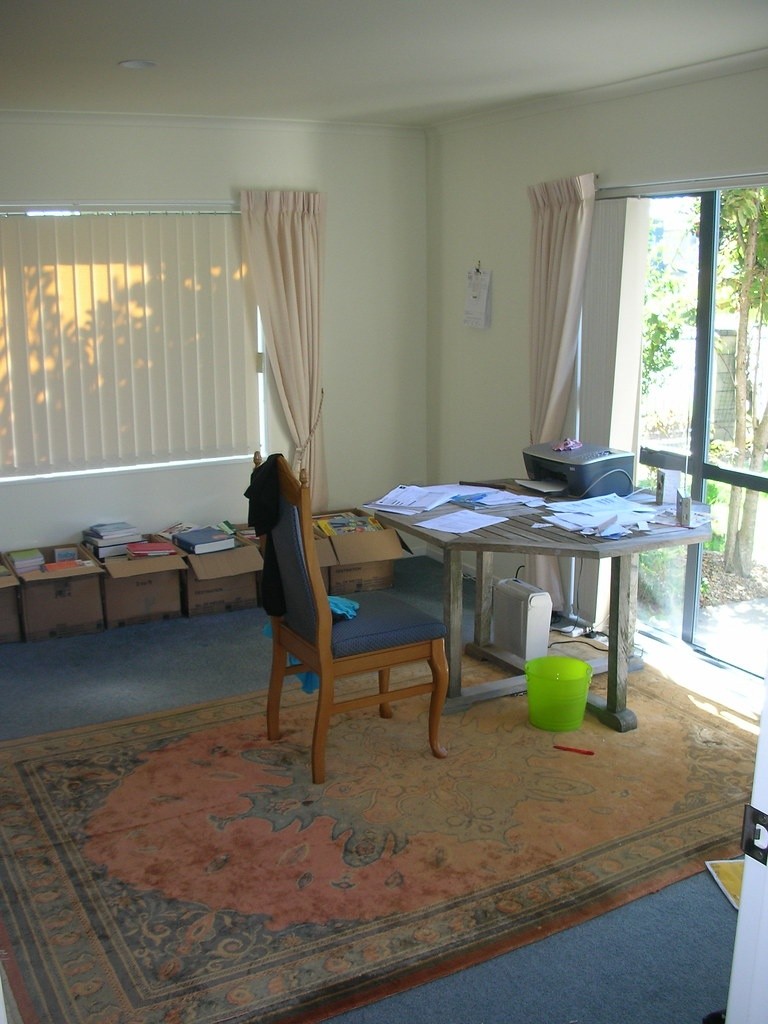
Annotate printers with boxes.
[518,439,635,497]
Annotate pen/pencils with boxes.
[553,742,596,758]
[458,477,508,491]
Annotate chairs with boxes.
[243,450,449,785]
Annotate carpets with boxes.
[0,630,761,1024]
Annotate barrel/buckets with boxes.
[525,657,592,732]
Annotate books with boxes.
[448,491,527,510]
[160,520,258,555]
[0,546,96,577]
[81,523,180,563]
[312,510,384,536]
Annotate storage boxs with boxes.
[156,534,264,619]
[79,534,189,630]
[0,553,21,645]
[5,543,106,643]
[311,508,414,596]
[232,524,340,596]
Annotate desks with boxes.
[372,477,713,734]
